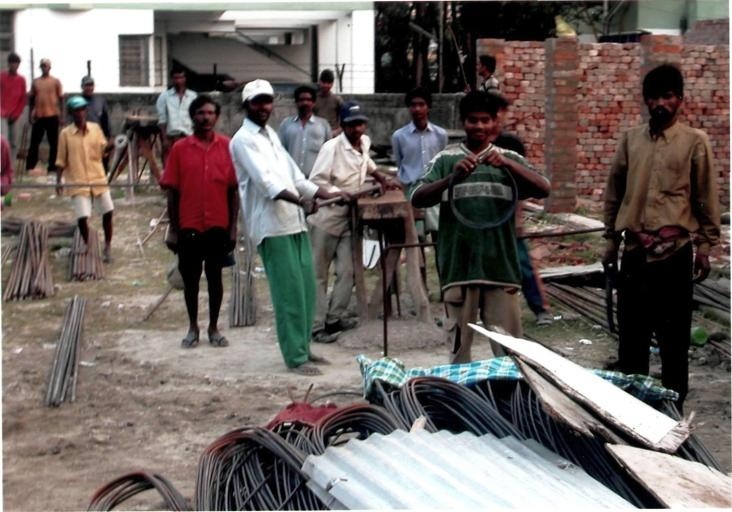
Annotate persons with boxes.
[78,76,111,175]
[407,93,552,363]
[230,79,353,374]
[156,70,241,349]
[603,65,721,417]
[1,132,15,197]
[55,94,116,264]
[25,59,66,174]
[1,52,27,148]
[279,67,447,343]
[487,94,562,325]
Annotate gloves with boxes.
[297,195,319,214]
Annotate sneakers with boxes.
[181,327,200,347]
[287,318,359,376]
[535,312,554,327]
[208,331,229,347]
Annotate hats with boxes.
[82,76,94,87]
[338,100,370,124]
[67,95,91,111]
[241,79,275,104]
[40,58,51,66]
